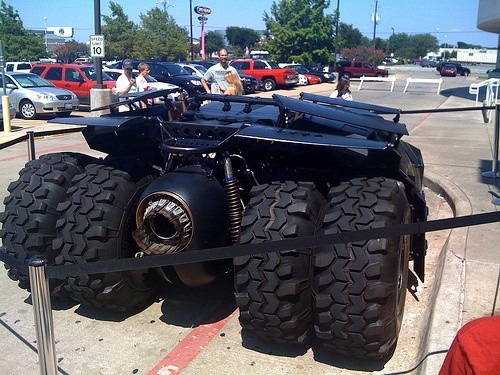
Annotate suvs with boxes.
[4,61,41,73]
[338,60,389,78]
[29,63,117,105]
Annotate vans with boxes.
[74,58,90,63]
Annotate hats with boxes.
[123,59,133,69]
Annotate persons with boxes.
[115,59,138,111]
[201,48,243,100]
[134,63,157,104]
[328,77,353,101]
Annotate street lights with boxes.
[44,17,48,52]
[391,28,394,53]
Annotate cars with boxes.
[0,72,80,119]
[414,60,471,77]
[101,59,336,97]
[89,68,189,105]
[486,69,496,74]
[39,56,62,64]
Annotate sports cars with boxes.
[0,88,429,360]
